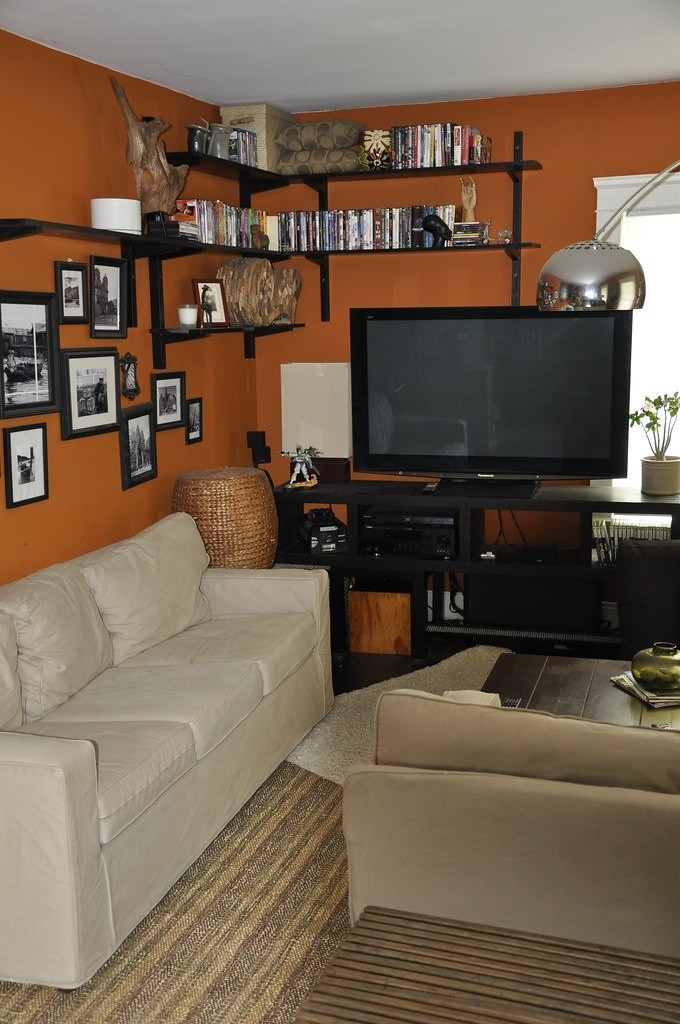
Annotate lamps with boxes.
[536,158,680,312]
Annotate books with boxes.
[178,200,267,248]
[391,122,492,169]
[277,205,482,252]
[592,519,671,568]
[610,671,680,708]
[230,128,258,168]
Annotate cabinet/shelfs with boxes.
[0,132,542,370]
[273,481,680,667]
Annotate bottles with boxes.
[185,124,210,153]
[208,123,232,159]
[631,642,680,693]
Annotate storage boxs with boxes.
[347,578,412,655]
[221,104,298,174]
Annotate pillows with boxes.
[276,119,367,176]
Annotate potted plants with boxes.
[630,391,680,496]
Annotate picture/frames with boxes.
[59,347,123,440]
[121,400,158,490]
[53,260,90,325]
[150,372,187,432]
[191,277,229,328]
[89,254,129,339]
[3,422,49,510]
[185,396,203,445]
[0,290,62,419]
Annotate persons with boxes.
[460,176,477,222]
[94,377,106,413]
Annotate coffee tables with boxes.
[482,653,680,729]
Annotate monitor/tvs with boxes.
[348,307,632,498]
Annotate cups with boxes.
[176,304,199,328]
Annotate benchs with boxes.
[291,906,680,1024]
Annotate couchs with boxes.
[342,689,680,959]
[0,511,334,995]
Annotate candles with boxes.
[178,303,199,330]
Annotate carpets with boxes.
[285,645,514,784]
